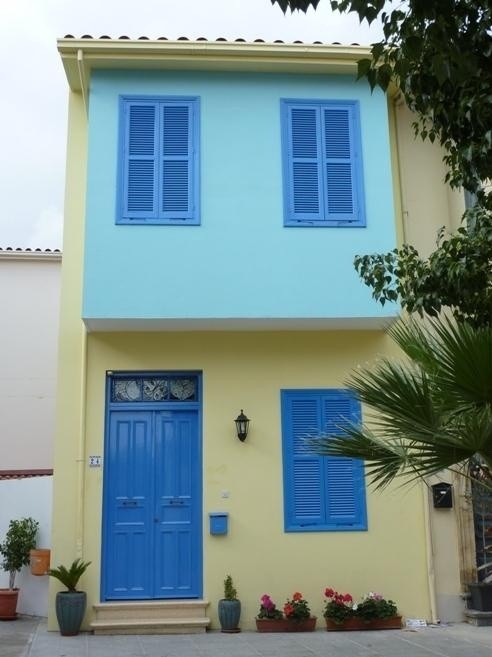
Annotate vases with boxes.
[468,582,491,611]
[255,614,401,633]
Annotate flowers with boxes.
[257,586,398,623]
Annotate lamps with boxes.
[235,409,250,443]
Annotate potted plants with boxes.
[44,557,92,635]
[215,575,244,632]
[0,515,40,617]
